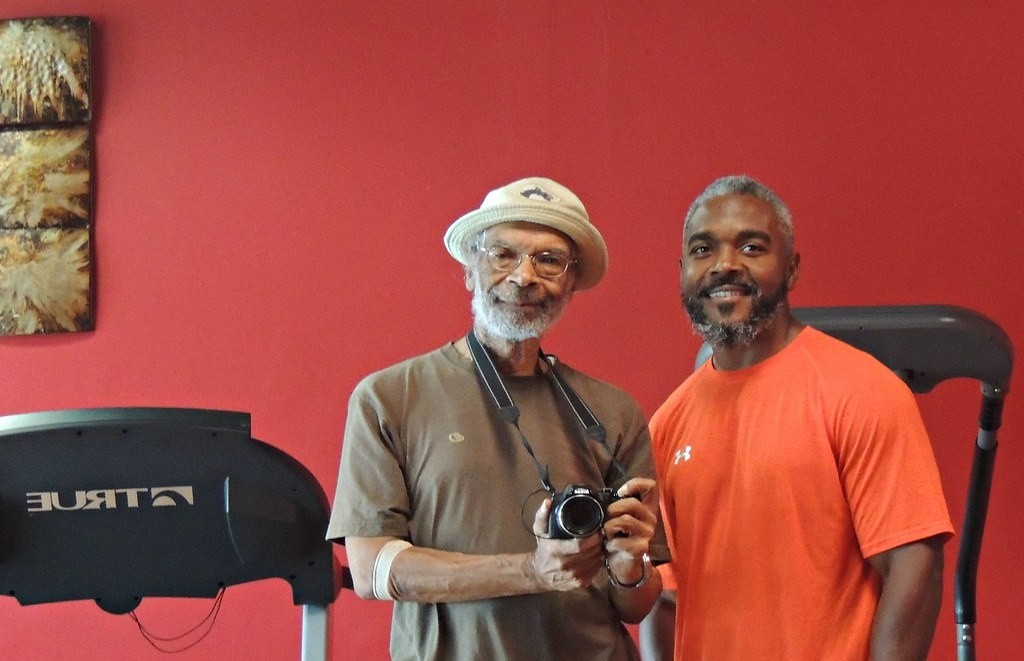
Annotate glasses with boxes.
[475,243,578,279]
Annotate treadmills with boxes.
[0,404,343,660]
[694,301,1018,661]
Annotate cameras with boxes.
[547,483,642,538]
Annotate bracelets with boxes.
[605,554,651,590]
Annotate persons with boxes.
[324,175,662,661]
[648,173,955,661]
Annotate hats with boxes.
[443,177,608,291]
